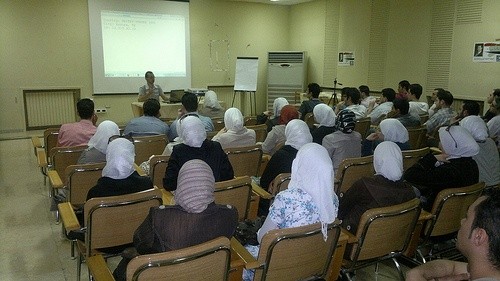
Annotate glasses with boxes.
[180,114,199,126]
[108,135,134,144]
[446,121,460,148]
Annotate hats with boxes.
[281,104,299,124]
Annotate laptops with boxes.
[163,89,184,103]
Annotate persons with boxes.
[298,83,321,119]
[336,80,500,209]
[211,108,256,149]
[242,142,339,281]
[169,93,214,137]
[138,71,169,102]
[322,111,361,174]
[338,141,416,231]
[123,98,169,135]
[262,104,298,153]
[57,98,97,147]
[310,103,337,145]
[163,113,234,191]
[113,159,239,281]
[77,138,153,255]
[78,120,120,164]
[257,119,314,217]
[353,188,500,281]
[201,90,227,118]
[257,97,289,132]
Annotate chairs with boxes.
[30,106,500,281]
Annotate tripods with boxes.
[327,80,339,107]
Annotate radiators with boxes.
[207,85,242,113]
[22,88,80,129]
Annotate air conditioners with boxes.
[267,51,308,114]
